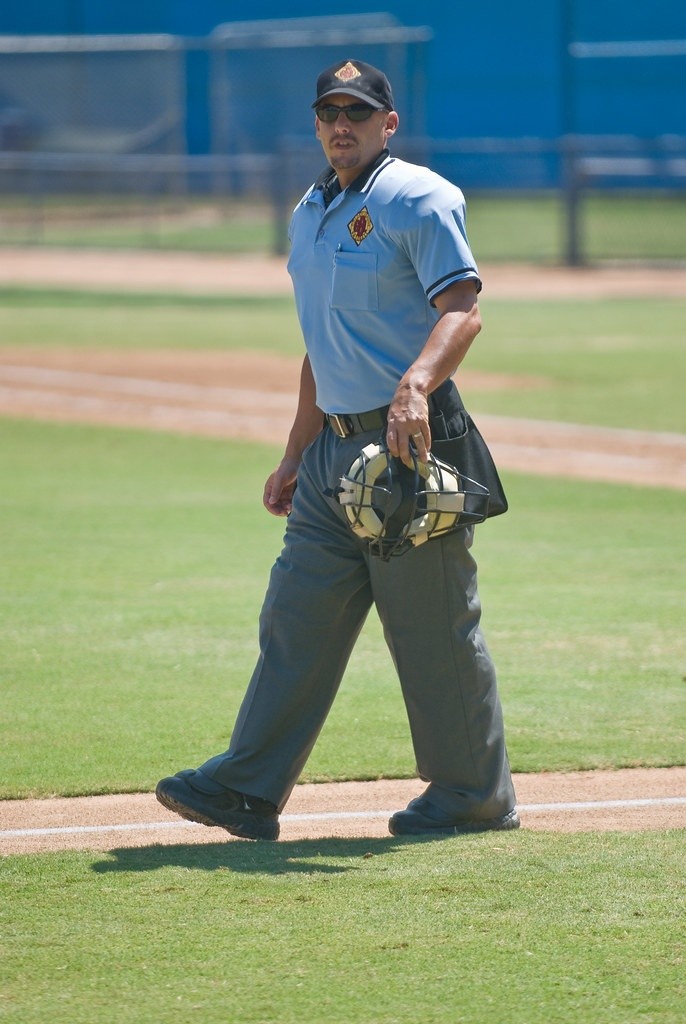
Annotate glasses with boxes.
[315,103,390,123]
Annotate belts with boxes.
[325,404,390,438]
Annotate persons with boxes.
[155,56,519,839]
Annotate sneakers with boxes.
[156,769,280,840]
[388,797,520,836]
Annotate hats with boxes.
[311,58,394,111]
